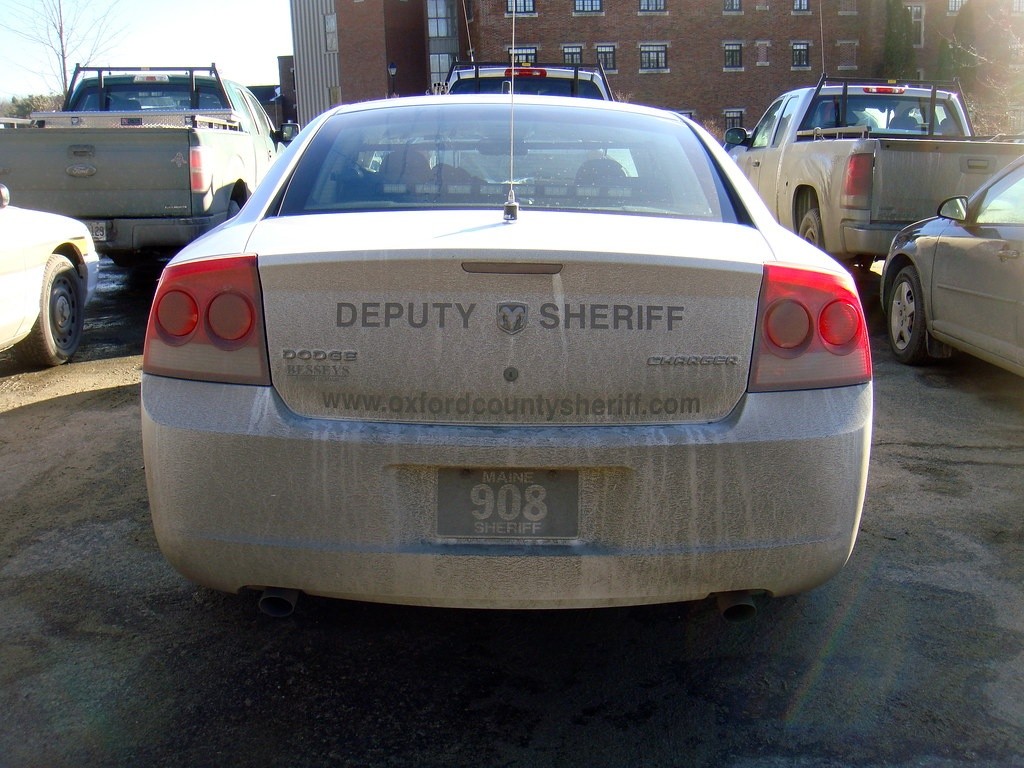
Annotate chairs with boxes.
[110,99,143,112]
[888,115,920,135]
[355,147,646,211]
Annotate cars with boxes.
[0,182,100,367]
[879,155,1024,384]
[138,94,875,621]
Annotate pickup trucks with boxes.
[723,73,1023,270]
[445,61,618,100]
[0,61,299,278]
